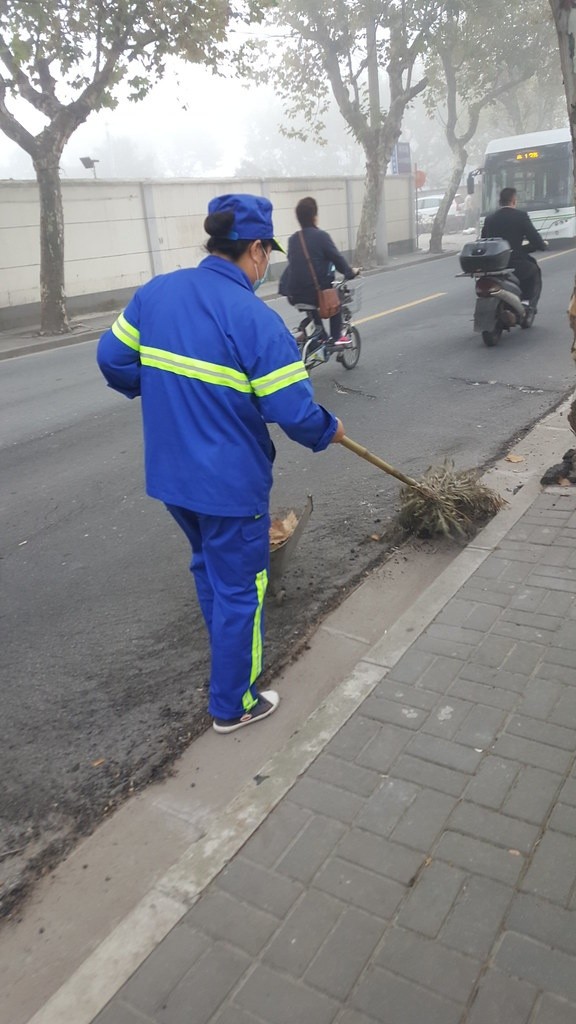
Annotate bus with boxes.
[466,127,576,239]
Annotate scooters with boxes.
[454,237,549,348]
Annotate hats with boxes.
[208,194,288,254]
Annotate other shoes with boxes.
[334,336,353,345]
[522,304,531,310]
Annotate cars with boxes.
[415,194,469,225]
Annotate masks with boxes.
[251,244,270,292]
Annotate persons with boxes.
[278,197,360,345]
[96,194,343,733]
[481,188,549,314]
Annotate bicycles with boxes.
[288,270,361,380]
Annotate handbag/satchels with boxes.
[318,289,341,319]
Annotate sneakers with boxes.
[213,690,280,733]
